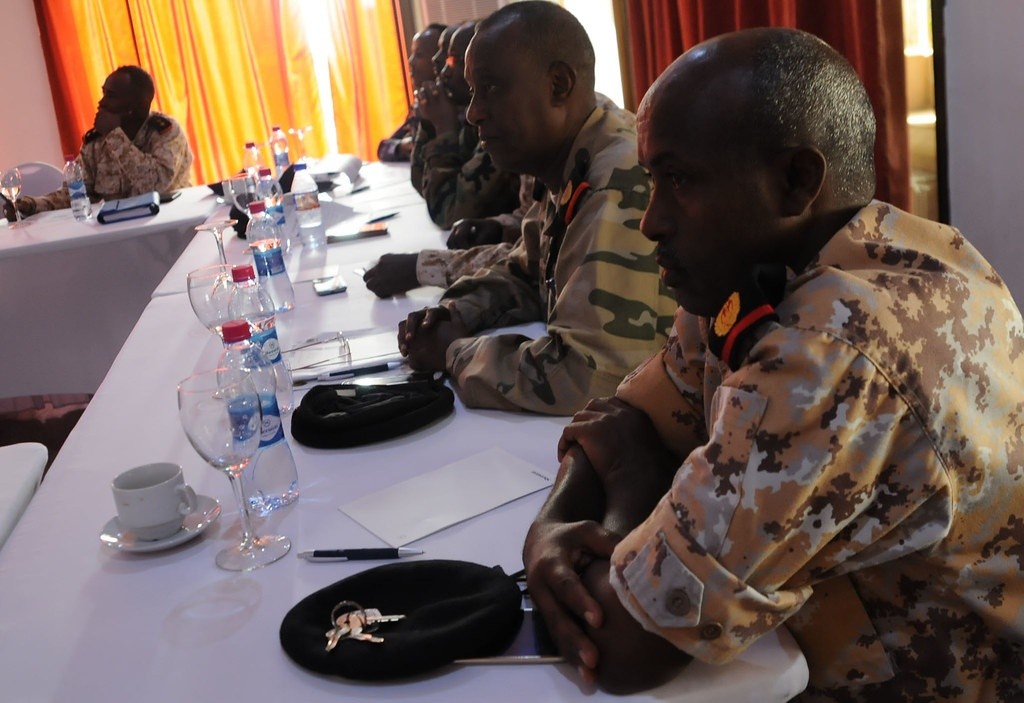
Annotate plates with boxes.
[99,494,221,554]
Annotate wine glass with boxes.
[0,167,31,230]
[187,219,238,399]
[177,368,291,572]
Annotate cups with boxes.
[222,173,256,219]
[111,461,199,541]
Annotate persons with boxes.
[361,21,619,298]
[523,25,1024,703]
[397,0,678,417]
[4,65,193,222]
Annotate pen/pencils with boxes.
[298,548,425,562]
[369,212,399,224]
[350,186,370,194]
[316,362,402,380]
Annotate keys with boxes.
[326,612,406,651]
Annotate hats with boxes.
[291,376,456,450]
[278,559,525,686]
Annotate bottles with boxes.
[246,164,323,312]
[243,127,288,180]
[217,264,300,517]
[63,155,93,223]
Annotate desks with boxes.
[0,157,811,703]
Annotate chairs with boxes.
[0,162,63,220]
